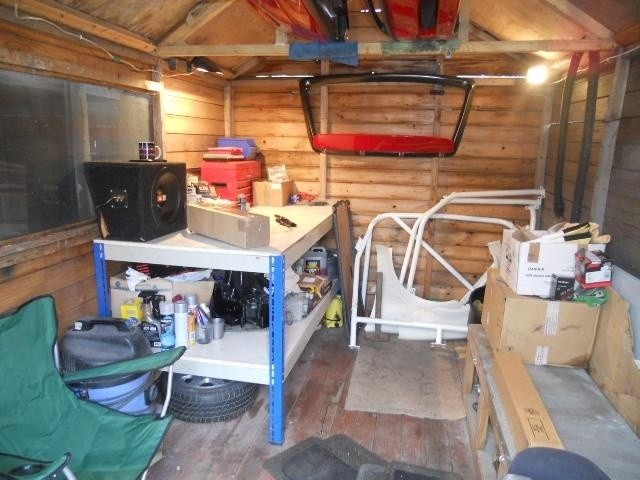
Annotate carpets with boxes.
[344,321,467,422]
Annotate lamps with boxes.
[189,56,224,76]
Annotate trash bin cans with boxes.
[464,284,486,326]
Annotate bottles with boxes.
[238,194,247,210]
[141,294,199,352]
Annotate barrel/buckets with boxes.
[303,246,328,276]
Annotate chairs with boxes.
[0,292,187,480]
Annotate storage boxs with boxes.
[252,178,294,207]
[480,267,603,369]
[186,200,270,249]
[491,352,565,455]
[497,227,606,296]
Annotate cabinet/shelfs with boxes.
[91,202,343,446]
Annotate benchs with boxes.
[460,322,640,480]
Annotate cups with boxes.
[195,323,214,344]
[138,142,161,161]
[212,318,225,340]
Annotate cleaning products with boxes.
[138,290,197,347]
